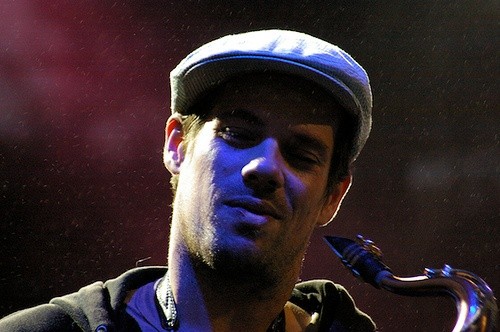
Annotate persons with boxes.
[3,27,381,332]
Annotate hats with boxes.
[169,28,372,171]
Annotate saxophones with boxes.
[324,232,500,332]
